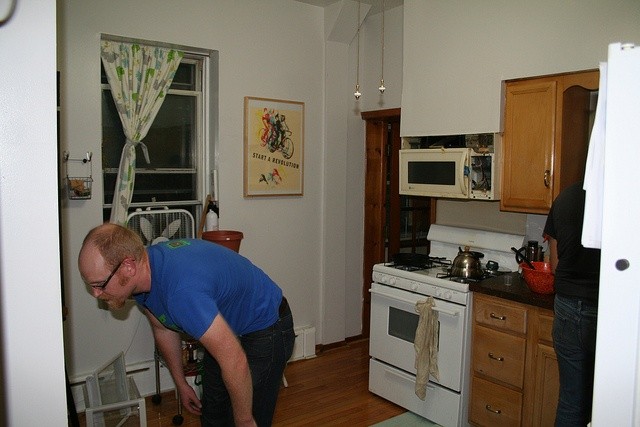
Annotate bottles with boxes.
[527,241,539,262]
[205,208,219,232]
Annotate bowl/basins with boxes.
[520,262,556,295]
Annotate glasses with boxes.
[85,257,134,287]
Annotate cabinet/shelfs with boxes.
[147,325,204,427]
[523,302,562,427]
[499,72,602,215]
[467,289,528,427]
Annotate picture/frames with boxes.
[243,95,305,198]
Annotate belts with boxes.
[236,296,287,338]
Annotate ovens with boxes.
[368,282,472,427]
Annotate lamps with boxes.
[354,84,362,99]
[378,80,387,94]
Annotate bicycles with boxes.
[268,129,294,159]
[258,125,287,147]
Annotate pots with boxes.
[391,252,447,266]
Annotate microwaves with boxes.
[397,147,471,199]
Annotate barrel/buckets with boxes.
[202,230,243,257]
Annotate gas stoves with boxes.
[371,257,482,306]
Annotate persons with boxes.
[79,222,295,426]
[542,160,603,426]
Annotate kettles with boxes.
[447,246,485,278]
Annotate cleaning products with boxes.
[204,202,218,232]
